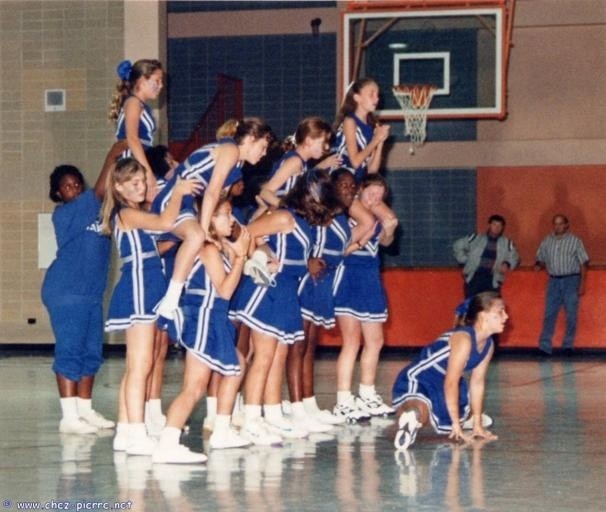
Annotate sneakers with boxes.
[114,433,335,481]
[394,449,416,482]
[462,414,493,429]
[60,393,419,463]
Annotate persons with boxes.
[105,59,400,465]
[40,140,130,436]
[453,214,519,360]
[532,212,589,358]
[393,291,512,449]
